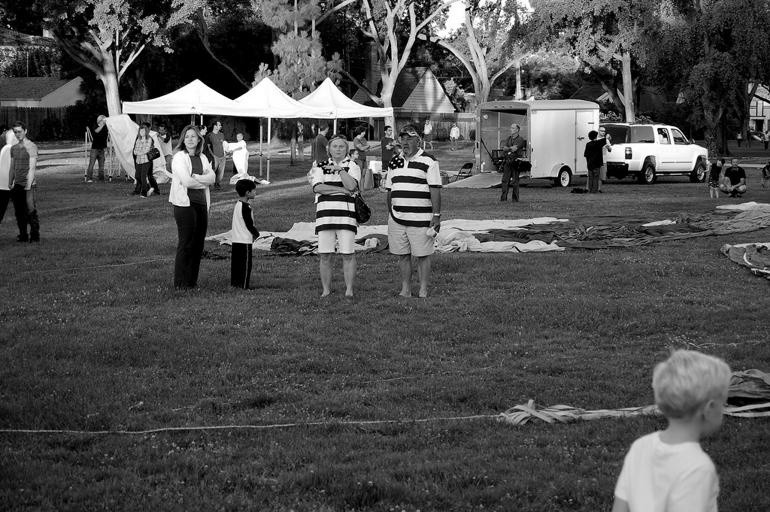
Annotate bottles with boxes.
[399,124,423,140]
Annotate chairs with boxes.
[147,187,155,197]
[418,290,428,298]
[97,174,104,181]
[174,279,185,290]
[399,290,412,297]
[139,195,148,199]
[132,190,141,194]
[87,177,93,183]
[16,234,29,243]
[153,189,160,195]
[30,234,40,244]
[189,283,200,293]
[214,183,221,191]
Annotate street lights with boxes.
[147,145,160,162]
[510,137,531,172]
[352,177,372,225]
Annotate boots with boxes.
[603,122,712,182]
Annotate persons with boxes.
[307,135,362,296]
[130,122,161,196]
[311,122,321,162]
[198,125,216,182]
[84,115,115,183]
[205,120,226,192]
[312,123,331,161]
[230,179,262,290]
[500,123,524,201]
[597,125,612,191]
[158,124,173,178]
[764,131,770,149]
[395,145,404,161]
[702,155,724,200]
[378,126,396,193]
[718,155,747,197]
[295,121,305,162]
[220,131,249,184]
[744,128,752,148]
[617,350,729,509]
[171,124,217,290]
[0,130,27,241]
[423,119,434,151]
[386,127,443,296]
[134,125,155,198]
[352,126,371,191]
[8,121,41,247]
[583,128,609,193]
[450,122,460,151]
[737,131,742,147]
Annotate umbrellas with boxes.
[598,189,602,194]
[512,198,519,202]
[501,197,508,202]
[584,188,589,193]
[737,195,742,198]
[728,194,736,198]
[344,289,354,296]
[320,290,331,297]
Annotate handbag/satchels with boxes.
[433,212,443,217]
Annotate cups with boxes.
[600,129,608,133]
